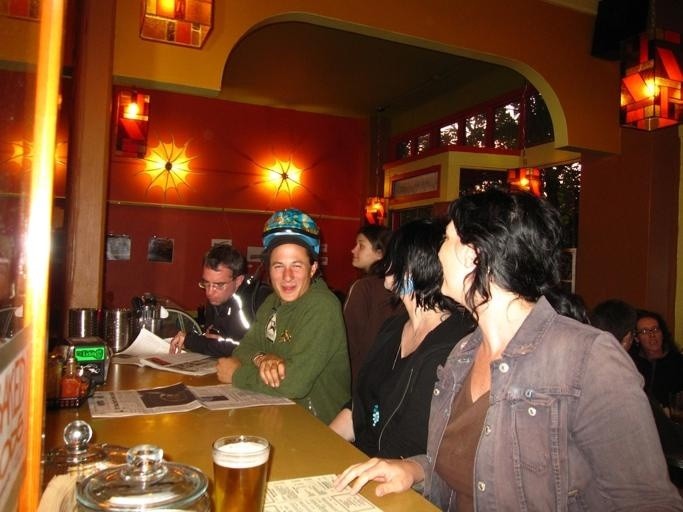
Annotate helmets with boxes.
[263,207,322,256]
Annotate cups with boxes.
[132,308,162,341]
[68,307,98,338]
[669,391,683,425]
[212,433,271,511]
[103,307,133,354]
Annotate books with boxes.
[110,327,218,377]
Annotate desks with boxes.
[47,328,442,512]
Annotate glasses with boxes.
[636,327,660,336]
[198,273,241,290]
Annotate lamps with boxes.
[4,120,68,178]
[362,194,388,226]
[615,24,682,131]
[505,162,543,200]
[257,153,305,200]
[112,84,151,160]
[137,0,217,53]
[130,130,202,203]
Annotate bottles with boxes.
[35,420,112,498]
[73,442,214,512]
[45,353,94,407]
